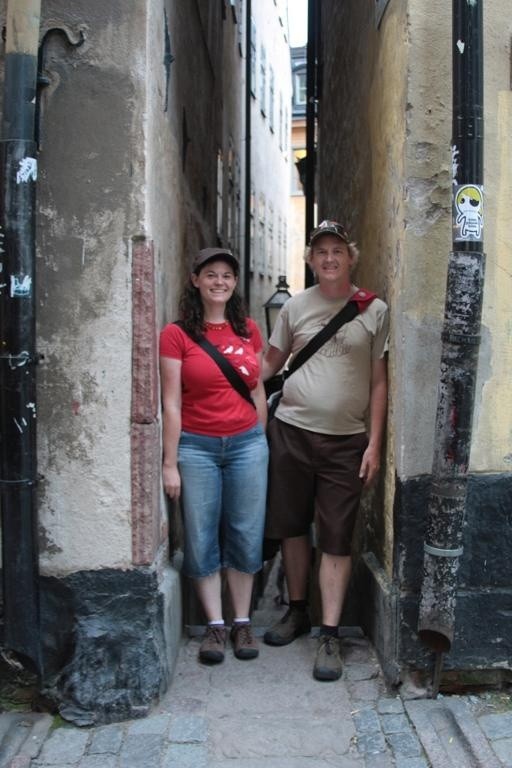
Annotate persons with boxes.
[261,220,389,681]
[160,247,270,662]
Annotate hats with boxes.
[310,220,350,243]
[192,248,239,271]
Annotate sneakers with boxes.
[230,619,259,660]
[313,634,342,682]
[199,624,226,664]
[264,608,311,647]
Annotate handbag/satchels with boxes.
[263,374,283,422]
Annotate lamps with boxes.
[263,275,291,338]
